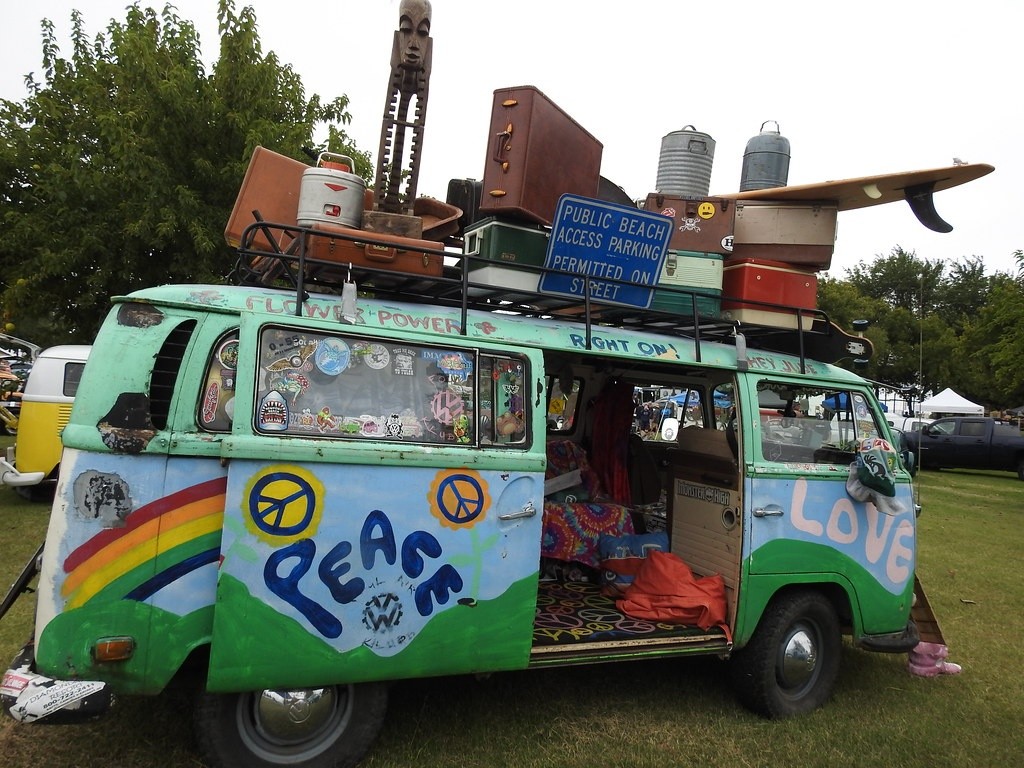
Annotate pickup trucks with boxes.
[897,416,1024,480]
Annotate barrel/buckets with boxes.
[656,125,717,196]
[740,120,790,192]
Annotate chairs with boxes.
[661,426,738,622]
[588,382,659,506]
[541,439,635,581]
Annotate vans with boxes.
[0,343,98,500]
[828,413,951,447]
[32,220,920,761]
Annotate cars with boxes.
[0,334,47,437]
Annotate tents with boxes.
[823,393,888,420]
[913,388,984,417]
[655,390,731,441]
[1006,405,1024,431]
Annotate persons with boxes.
[634,400,660,433]
